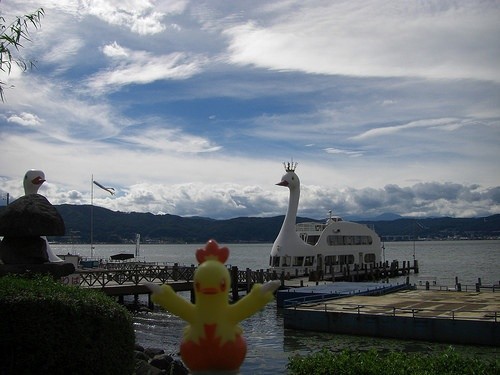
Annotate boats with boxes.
[267,161,383,277]
[0,170,65,263]
